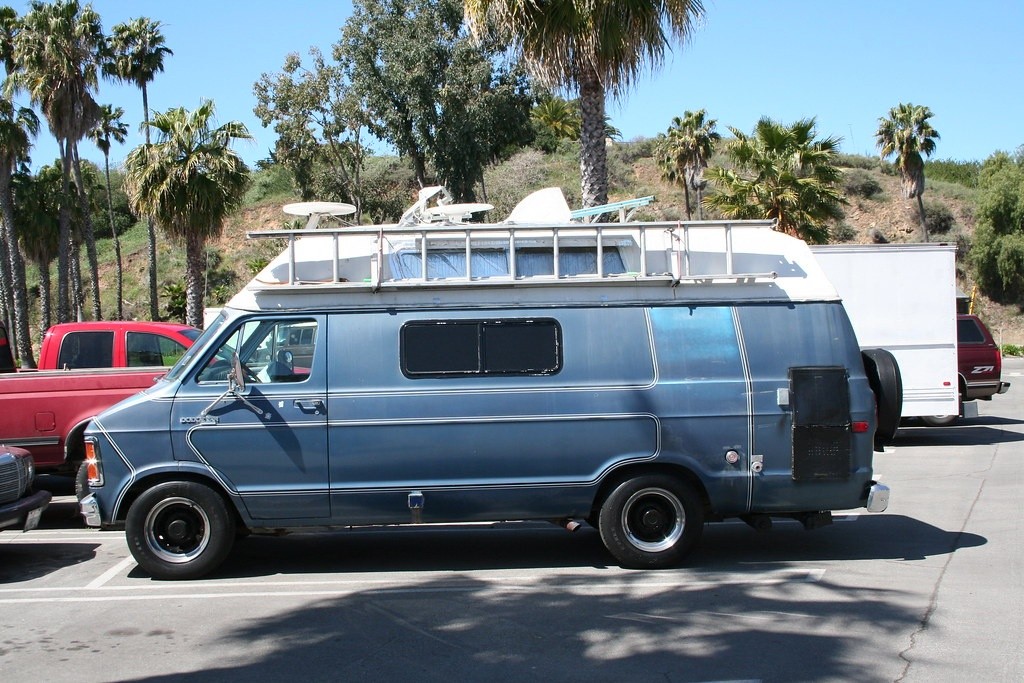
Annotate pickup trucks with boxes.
[0,324,207,509]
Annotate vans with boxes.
[76,180,904,584]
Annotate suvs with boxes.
[900,314,1010,428]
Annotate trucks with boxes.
[802,238,960,430]
[28,320,313,382]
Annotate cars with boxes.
[0,443,53,532]
